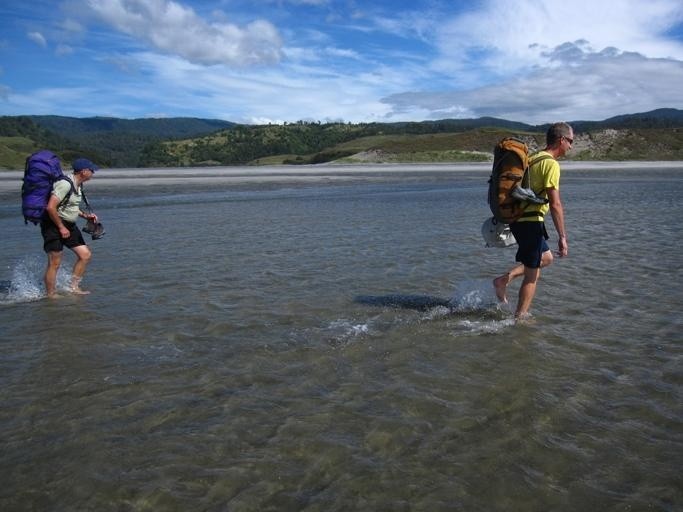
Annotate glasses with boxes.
[555,135,573,145]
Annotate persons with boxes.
[38,158,99,298]
[492,120,574,321]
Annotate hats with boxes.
[72,157,101,172]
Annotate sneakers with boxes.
[81,220,93,234]
[91,223,105,240]
[484,222,519,249]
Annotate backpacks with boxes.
[487,136,556,225]
[21,150,90,226]
